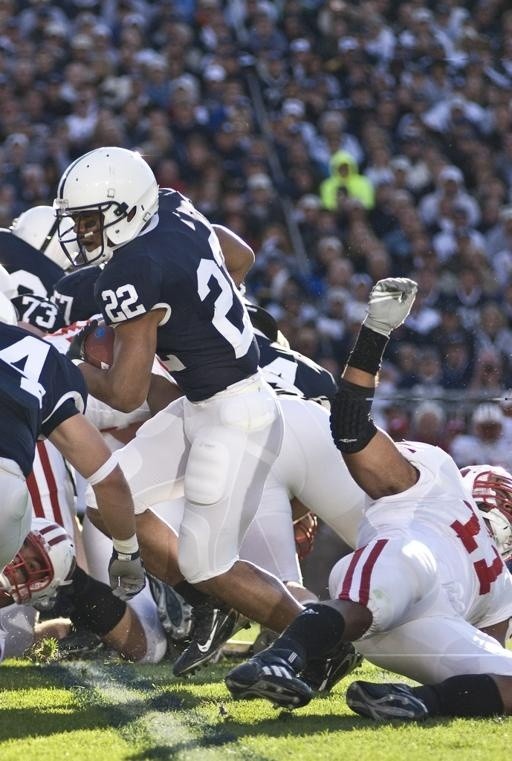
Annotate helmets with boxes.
[9,146,159,271]
[0,517,77,611]
[459,465,512,563]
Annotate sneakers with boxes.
[150,573,252,679]
[225,625,429,721]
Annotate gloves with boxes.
[65,320,98,361]
[108,538,146,601]
[363,277,419,339]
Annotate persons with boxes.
[224,277,512,722]
[0,143,377,707]
[0,0,512,471]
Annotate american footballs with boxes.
[82,324,116,371]
